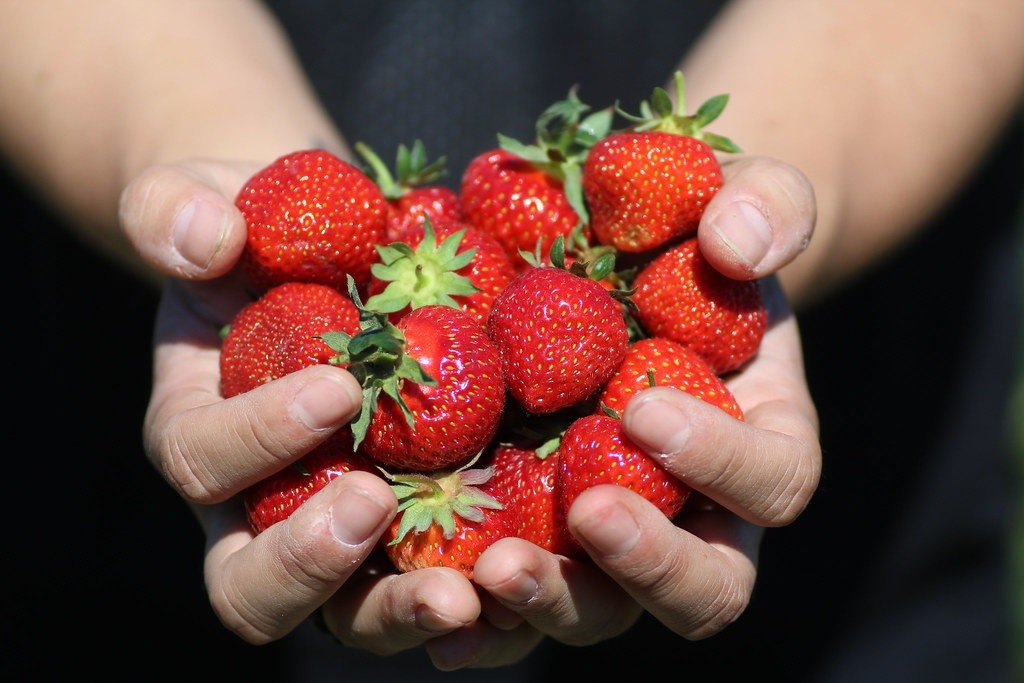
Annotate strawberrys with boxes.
[217,67,768,586]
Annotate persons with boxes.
[0,0,1024,672]
